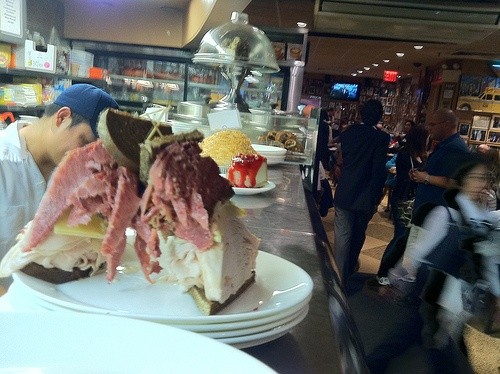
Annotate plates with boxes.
[219,173,276,195]
[252,144,288,165]
[0,226,315,374]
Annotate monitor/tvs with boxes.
[330,81,361,102]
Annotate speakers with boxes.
[325,75,331,84]
[365,78,370,87]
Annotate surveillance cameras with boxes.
[414,63,421,66]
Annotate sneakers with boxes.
[376,275,390,285]
[389,268,416,282]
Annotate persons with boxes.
[0,82,122,299]
[331,99,390,289]
[313,99,500,374]
[401,106,475,283]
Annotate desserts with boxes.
[0,108,262,314]
[227,154,268,188]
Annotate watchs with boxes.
[423,172,430,185]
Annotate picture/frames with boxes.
[457,115,500,151]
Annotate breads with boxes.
[259,130,304,152]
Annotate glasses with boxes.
[428,120,446,128]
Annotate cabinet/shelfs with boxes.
[0,68,107,115]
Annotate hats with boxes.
[53,83,120,138]
